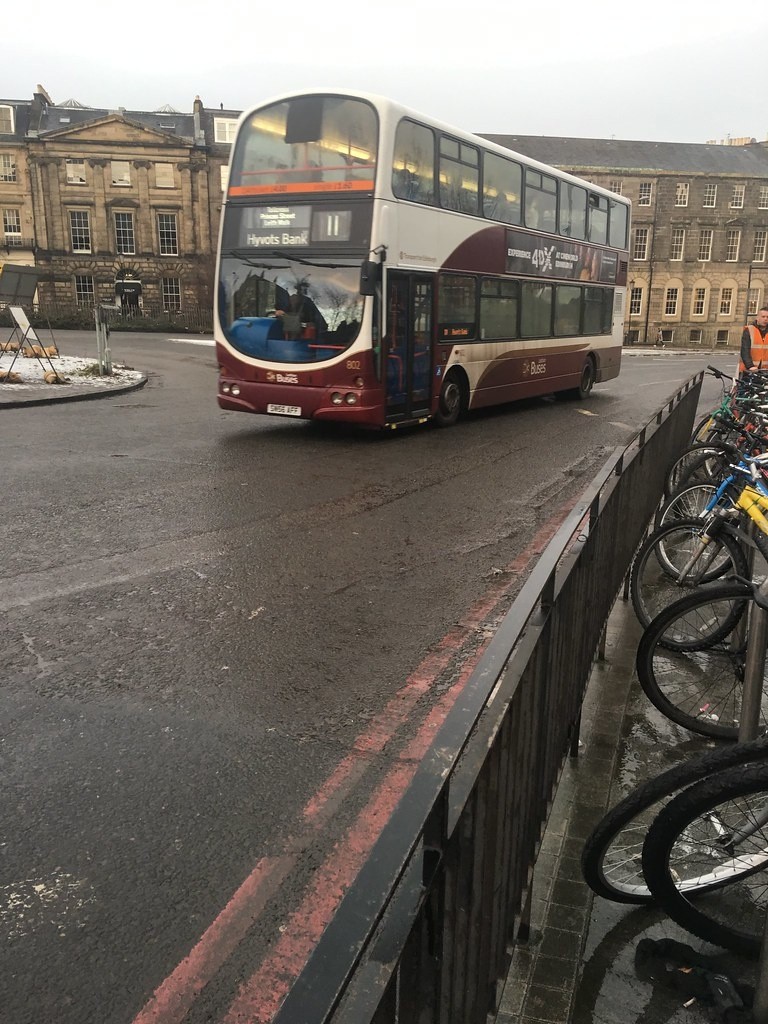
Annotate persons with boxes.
[526,194,540,229]
[653,328,665,347]
[740,307,768,370]
[485,191,513,224]
[274,268,323,328]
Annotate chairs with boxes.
[393,172,538,228]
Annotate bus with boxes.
[211,85,634,433]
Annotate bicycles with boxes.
[581,359,768,959]
